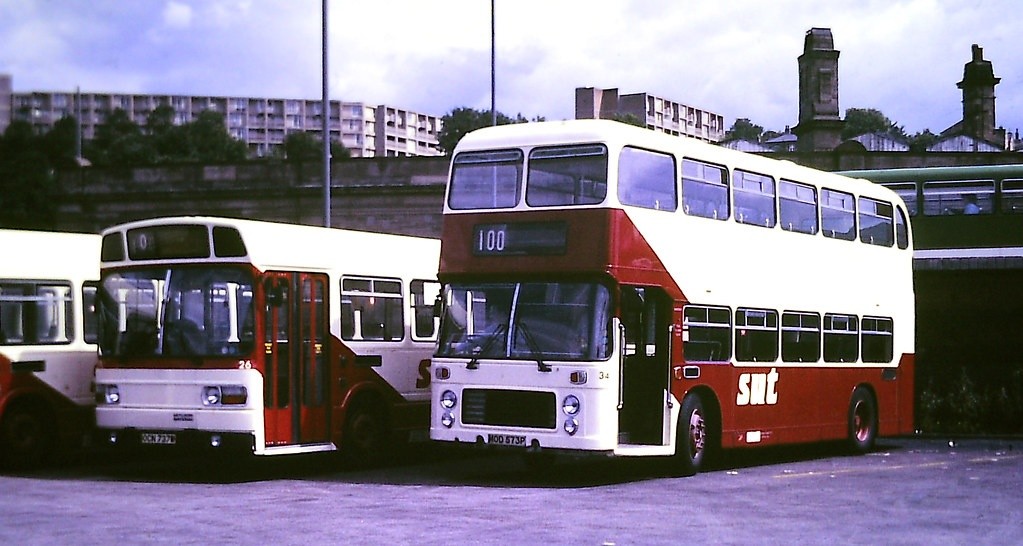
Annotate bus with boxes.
[429,121,919,474]
[0,228,261,474]
[94,214,495,473]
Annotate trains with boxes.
[821,165,1023,249]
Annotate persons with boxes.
[957,192,983,216]
